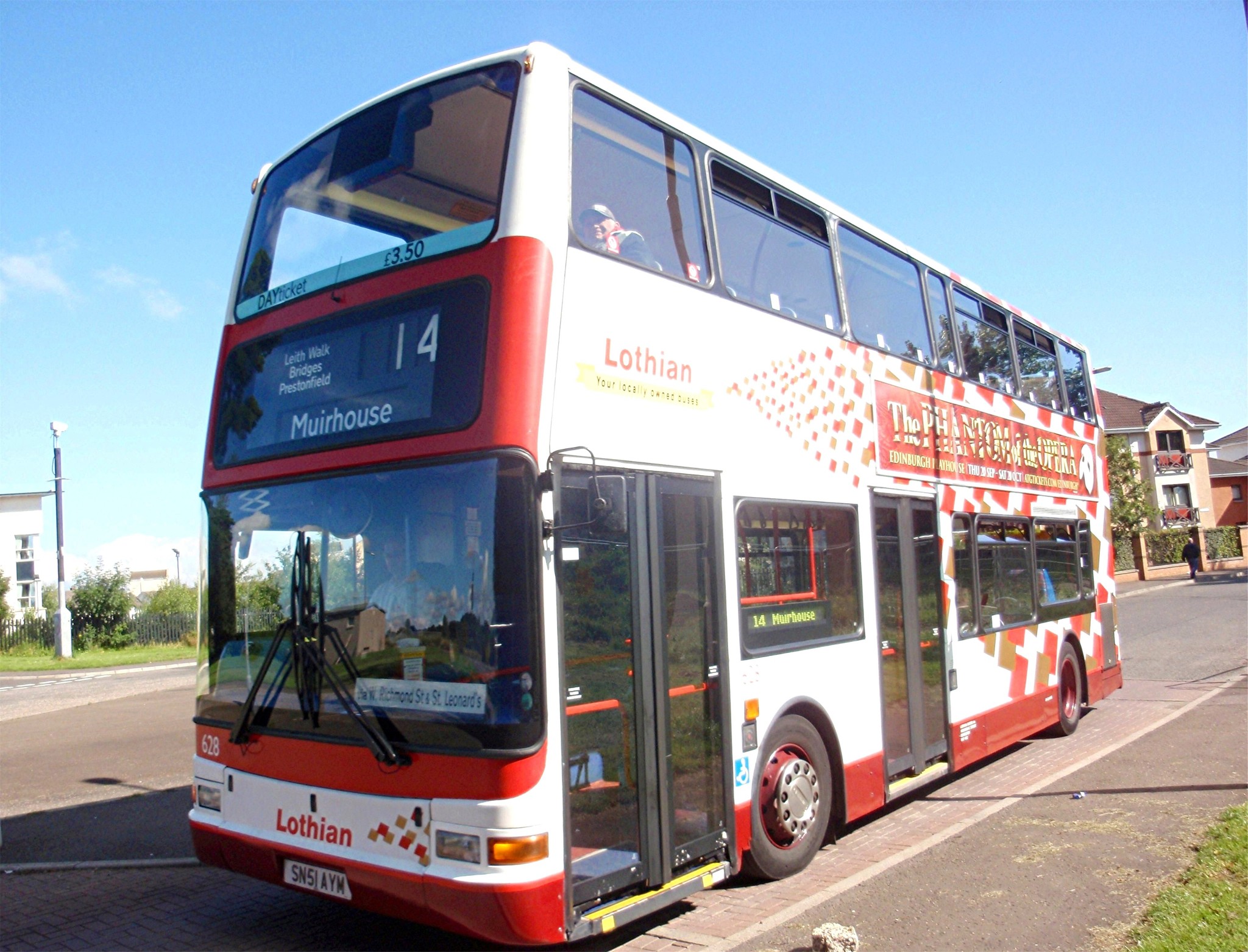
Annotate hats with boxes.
[578,204,614,222]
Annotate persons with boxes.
[578,202,663,273]
[1180,537,1200,580]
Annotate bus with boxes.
[186,42,1122,949]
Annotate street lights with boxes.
[171,548,180,583]
[50,420,72,659]
